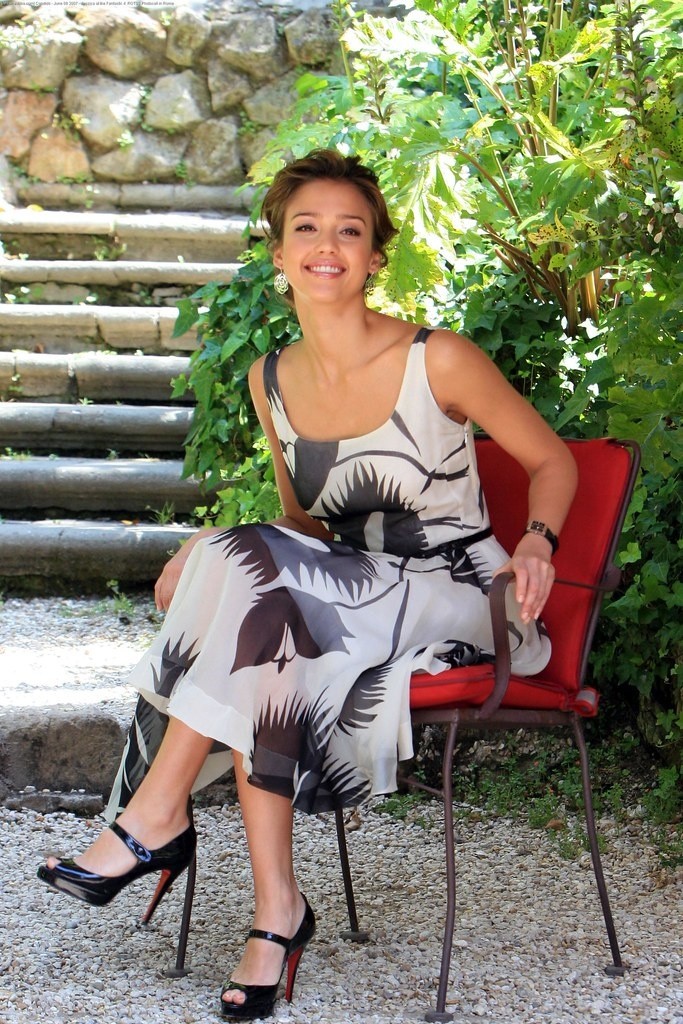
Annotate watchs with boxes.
[522,520,559,556]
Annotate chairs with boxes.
[164,438,641,1024]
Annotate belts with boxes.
[419,527,494,559]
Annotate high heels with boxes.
[221,892,316,1018]
[37,819,198,925]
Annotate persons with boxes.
[36,149,577,1021]
